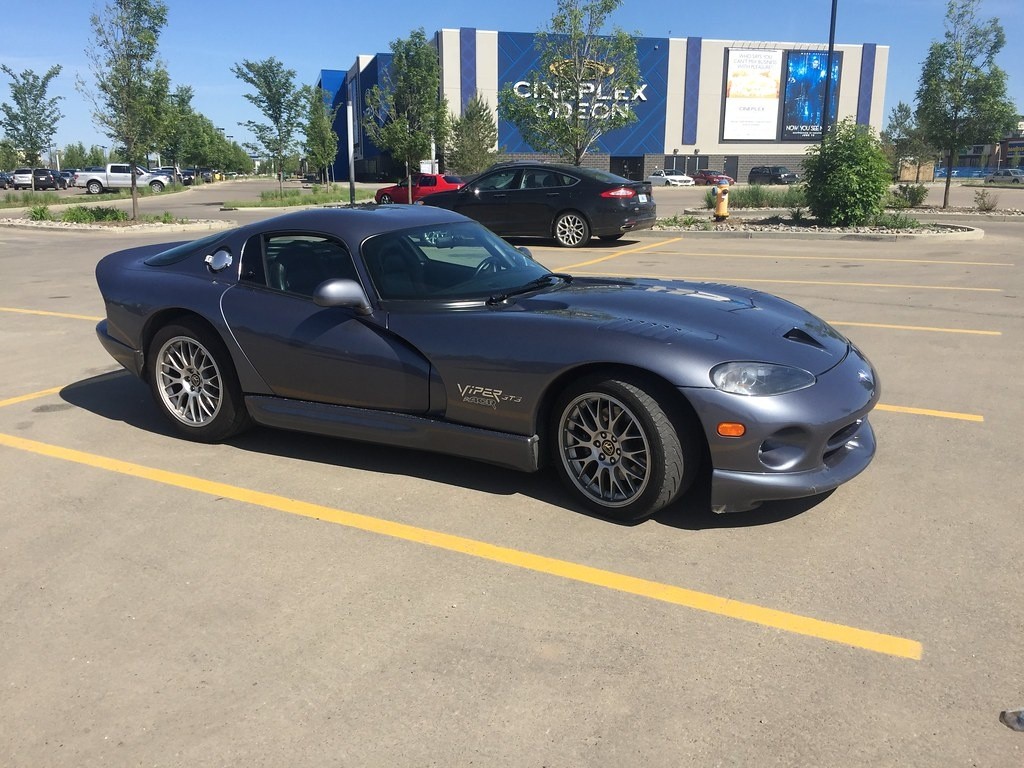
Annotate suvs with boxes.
[12,167,33,190]
[31,168,68,191]
[748,164,800,185]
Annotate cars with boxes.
[59,171,74,187]
[152,164,248,186]
[646,168,696,187]
[984,168,1024,185]
[412,160,658,249]
[690,168,734,187]
[62,166,106,180]
[934,166,1024,177]
[375,172,466,204]
[0,170,15,190]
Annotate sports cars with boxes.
[95,201,879,524]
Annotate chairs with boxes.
[371,241,428,294]
[270,245,326,295]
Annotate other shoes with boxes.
[1004,710,1024,732]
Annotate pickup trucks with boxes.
[73,163,170,195]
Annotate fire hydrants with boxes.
[711,178,730,222]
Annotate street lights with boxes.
[226,136,233,143]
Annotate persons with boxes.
[805,59,825,124]
